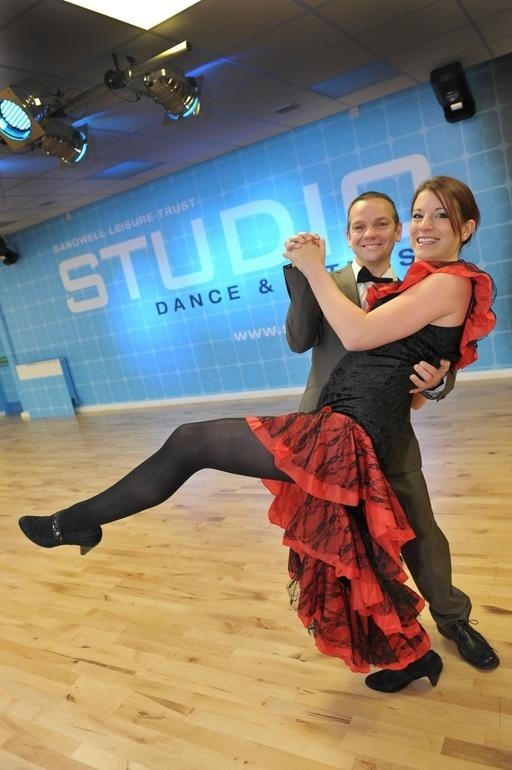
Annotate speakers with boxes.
[0,235,18,265]
[430,61,476,123]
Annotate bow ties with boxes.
[353,265,394,286]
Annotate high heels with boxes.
[17,510,104,558]
[362,648,443,694]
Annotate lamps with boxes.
[0,41,201,167]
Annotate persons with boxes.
[277,189,504,672]
[14,174,498,694]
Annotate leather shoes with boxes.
[435,619,501,671]
